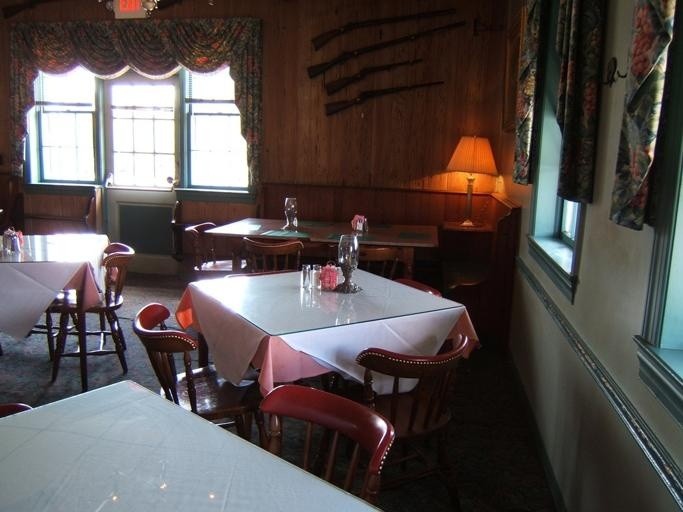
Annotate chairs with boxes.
[396,279,442,297]
[259,384,396,506]
[185,222,247,280]
[0,403,33,418]
[45,242,136,382]
[328,243,402,280]
[243,237,304,273]
[0,402,33,419]
[133,302,270,451]
[22,285,84,359]
[308,333,469,512]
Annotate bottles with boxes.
[7,234,20,253]
[301,265,322,290]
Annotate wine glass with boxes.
[334,234,364,293]
[281,198,300,232]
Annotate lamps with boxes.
[445,136,499,227]
[97,0,160,19]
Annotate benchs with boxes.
[256,182,521,347]
